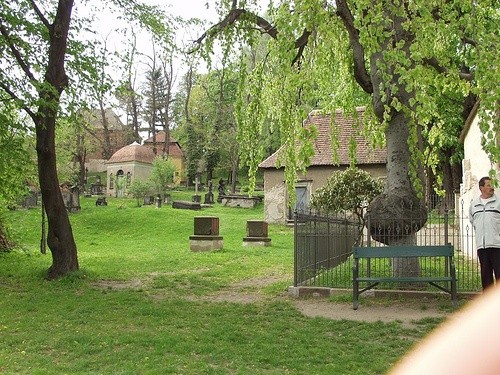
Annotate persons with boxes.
[468,176,500,292]
